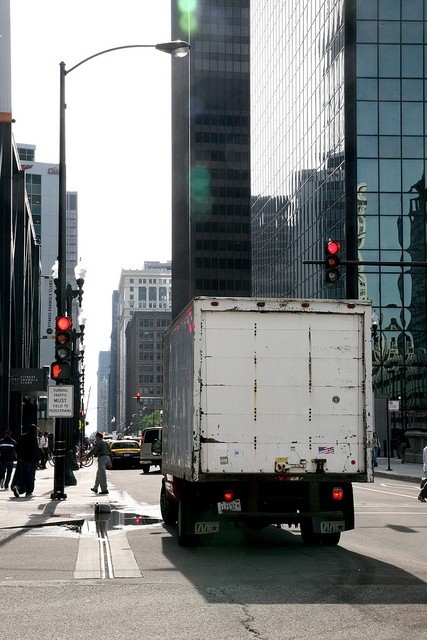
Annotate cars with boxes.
[109,440,141,469]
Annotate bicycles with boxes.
[75,448,93,467]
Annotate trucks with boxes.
[162,295,374,548]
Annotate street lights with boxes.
[69,309,86,471]
[52,255,86,485]
[52,41,191,500]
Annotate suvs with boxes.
[140,427,163,474]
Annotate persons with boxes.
[36,432,45,470]
[40,432,49,469]
[80,432,112,494]
[11,423,42,498]
[418,446,427,502]
[372,432,381,466]
[0,429,18,489]
[397,433,409,464]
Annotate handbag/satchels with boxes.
[106,456,113,469]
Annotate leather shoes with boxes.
[99,490,108,494]
[91,488,98,493]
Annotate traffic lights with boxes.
[50,361,72,381]
[55,314,71,357]
[323,240,342,285]
[137,393,140,402]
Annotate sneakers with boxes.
[4,481,8,489]
[0,479,4,489]
[26,493,37,498]
[12,486,20,499]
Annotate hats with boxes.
[44,431,50,435]
